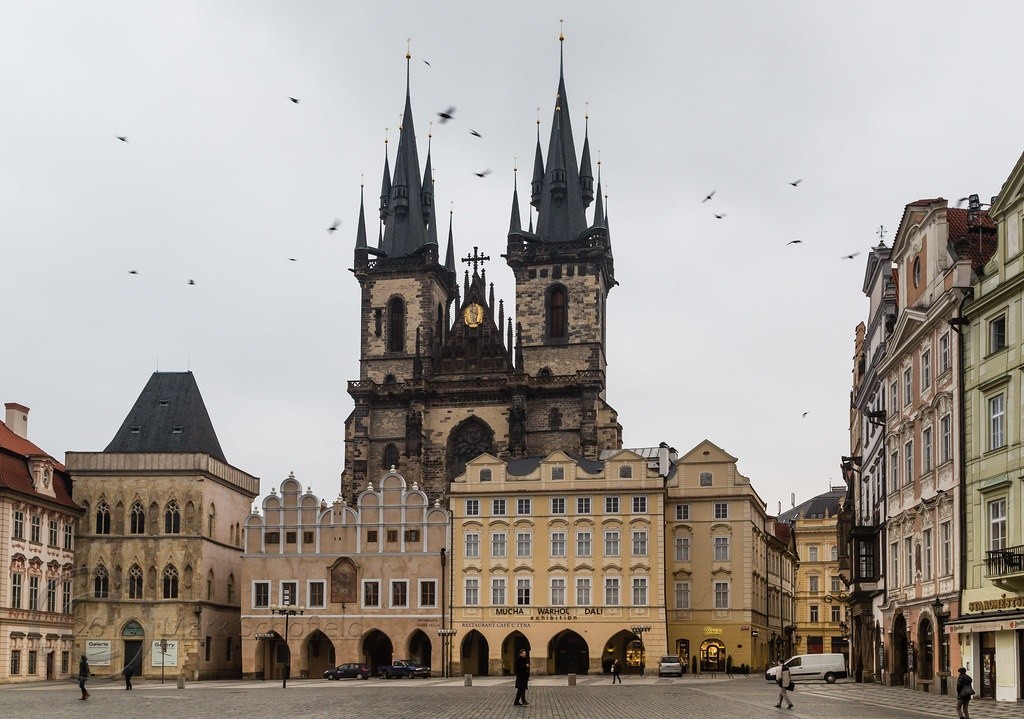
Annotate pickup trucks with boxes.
[377,658,430,679]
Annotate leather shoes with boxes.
[787,704,793,709]
[775,705,781,708]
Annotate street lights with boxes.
[269,602,308,689]
[631,623,652,677]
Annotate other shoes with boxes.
[514,699,529,706]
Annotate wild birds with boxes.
[107,47,863,423]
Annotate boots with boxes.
[78,692,90,700]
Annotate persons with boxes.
[956,668,973,719]
[122,661,133,690]
[775,658,794,709]
[613,658,621,684]
[78,655,91,701]
[514,649,530,707]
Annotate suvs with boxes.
[323,662,369,681]
[660,655,684,676]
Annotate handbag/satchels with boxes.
[785,682,794,691]
[958,684,975,699]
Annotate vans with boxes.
[765,652,848,685]
